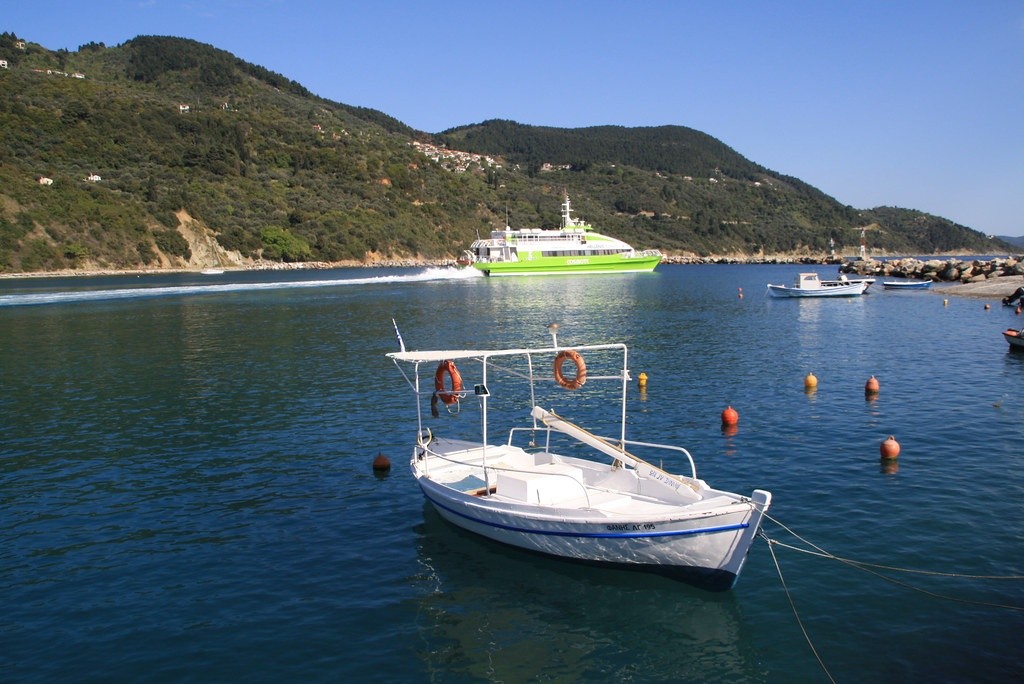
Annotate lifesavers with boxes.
[436,359,463,403]
[554,348,586,389]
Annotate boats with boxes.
[767,278,875,297]
[384,317,772,593]
[464,187,666,277]
[883,280,933,290]
[1002,328,1024,348]
